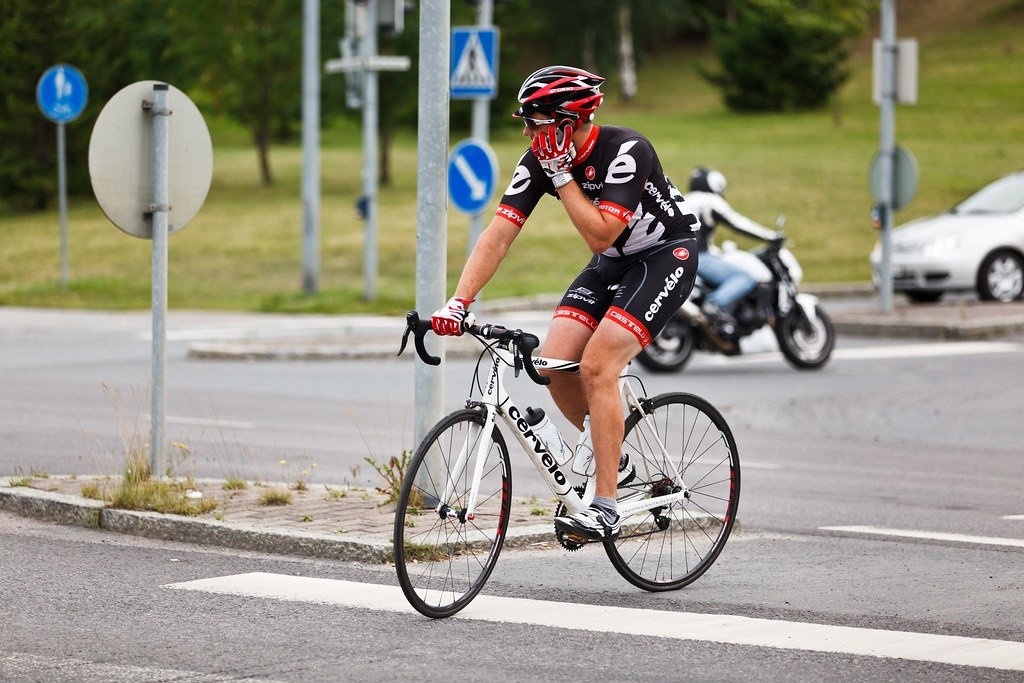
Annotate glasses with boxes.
[521,114,564,129]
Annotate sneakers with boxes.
[616,453,636,488]
[554,507,622,540]
[702,301,737,327]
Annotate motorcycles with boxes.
[635,210,834,375]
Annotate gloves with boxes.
[532,124,577,177]
[429,297,476,338]
[777,231,784,242]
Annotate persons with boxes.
[675,165,786,336]
[431,65,699,540]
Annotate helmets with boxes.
[511,65,608,128]
[691,165,727,197]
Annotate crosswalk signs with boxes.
[448,26,501,98]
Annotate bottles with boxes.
[572,408,596,476]
[525,406,574,467]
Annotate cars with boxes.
[870,167,1023,303]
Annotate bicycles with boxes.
[393,310,746,619]
